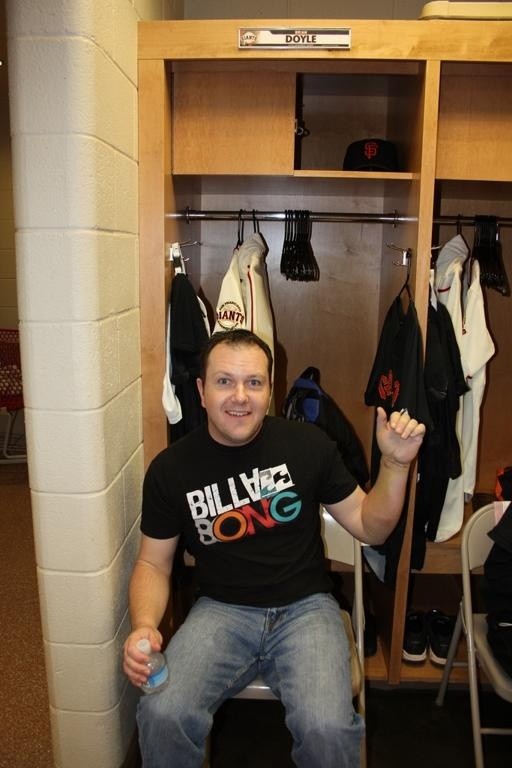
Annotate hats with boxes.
[343,139,400,172]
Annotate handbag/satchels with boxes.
[364,248,421,419]
[281,366,370,489]
[484,543,512,677]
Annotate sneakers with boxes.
[364,611,377,657]
[401,608,455,665]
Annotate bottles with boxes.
[133,637,170,694]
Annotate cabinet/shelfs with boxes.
[136,16,512,685]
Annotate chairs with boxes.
[201,508,366,766]
[436,500,511,768]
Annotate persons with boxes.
[482,501,510,679]
[122,331,428,768]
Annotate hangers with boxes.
[280,209,320,282]
[456,213,462,235]
[234,209,243,250]
[470,214,511,297]
[173,241,187,277]
[253,208,258,234]
[396,256,414,304]
